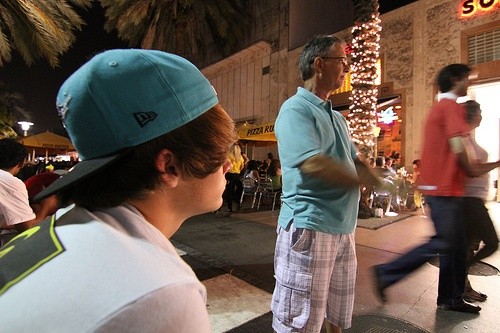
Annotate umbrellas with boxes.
[17,131,73,150]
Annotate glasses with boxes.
[309,55,348,64]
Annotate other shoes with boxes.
[211,208,231,216]
[228,201,232,211]
[237,203,240,210]
[462,288,487,301]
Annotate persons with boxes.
[0,49,238,333]
[0,138,80,234]
[367,63,500,313]
[271,34,406,333]
[214,140,424,218]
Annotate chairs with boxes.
[370,186,395,213]
[239,169,281,212]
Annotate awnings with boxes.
[332,95,400,112]
[232,118,277,142]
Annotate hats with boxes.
[30,47,219,202]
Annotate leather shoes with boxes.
[366,262,388,305]
[437,296,482,312]
